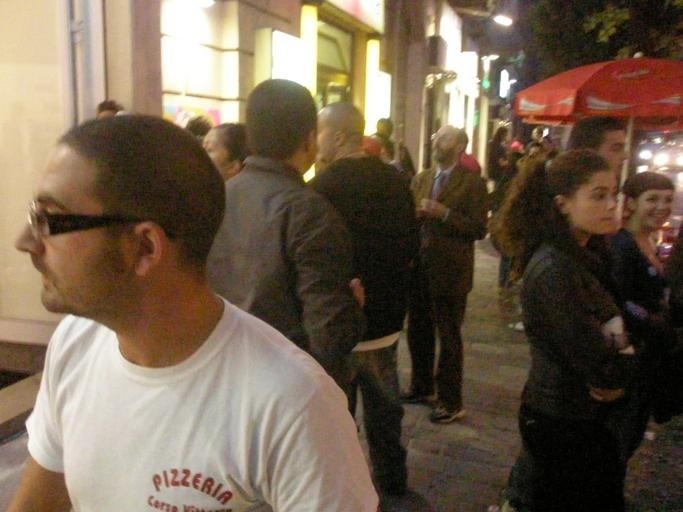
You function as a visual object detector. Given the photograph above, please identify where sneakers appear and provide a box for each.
[429,404,466,423]
[400,384,437,403]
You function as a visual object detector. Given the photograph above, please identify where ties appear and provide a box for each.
[432,172,444,200]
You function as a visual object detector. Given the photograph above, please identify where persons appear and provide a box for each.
[560,115,627,178]
[492,146,631,510]
[393,121,489,426]
[597,171,675,454]
[0,115,384,512]
[199,80,367,413]
[301,98,423,494]
[91,97,554,298]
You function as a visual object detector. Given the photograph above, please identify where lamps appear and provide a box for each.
[493,15,514,28]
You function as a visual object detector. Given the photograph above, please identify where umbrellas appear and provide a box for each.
[510,48,683,220]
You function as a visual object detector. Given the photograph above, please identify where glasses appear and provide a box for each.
[27,200,179,241]
